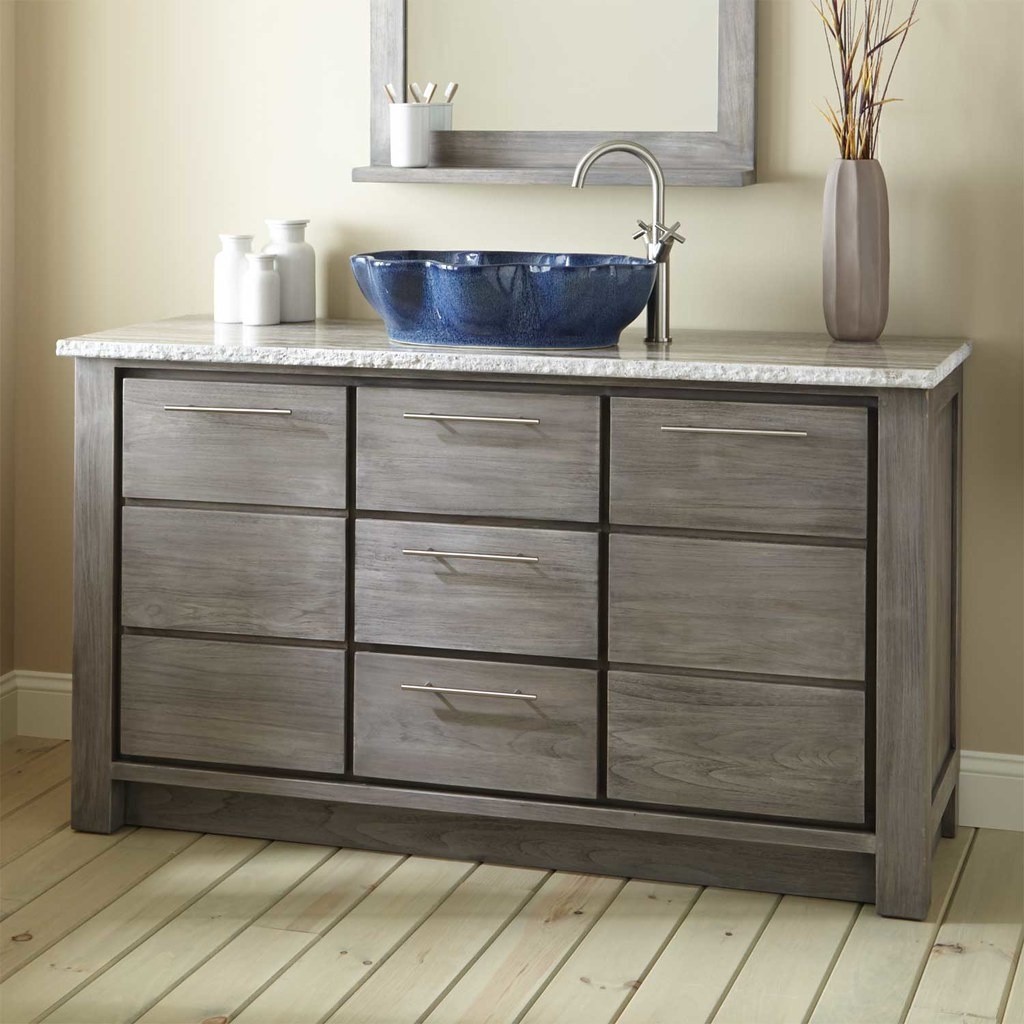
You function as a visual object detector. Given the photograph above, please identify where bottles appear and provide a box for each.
[260,219,316,323]
[213,234,254,323]
[239,253,281,326]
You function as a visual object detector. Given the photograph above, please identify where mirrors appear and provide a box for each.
[350,0,755,189]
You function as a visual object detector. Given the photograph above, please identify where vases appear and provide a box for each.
[823,158,890,341]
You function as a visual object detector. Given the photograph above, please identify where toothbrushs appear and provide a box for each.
[444,81,459,103]
[383,82,397,103]
[407,82,423,104]
[423,81,438,105]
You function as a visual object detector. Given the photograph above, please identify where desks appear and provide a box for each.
[54,312,975,919]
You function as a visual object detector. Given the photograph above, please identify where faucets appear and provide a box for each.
[568,138,685,345]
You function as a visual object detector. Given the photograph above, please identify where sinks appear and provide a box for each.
[348,250,659,349]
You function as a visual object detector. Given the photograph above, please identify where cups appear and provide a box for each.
[390,102,429,168]
[429,103,453,130]
